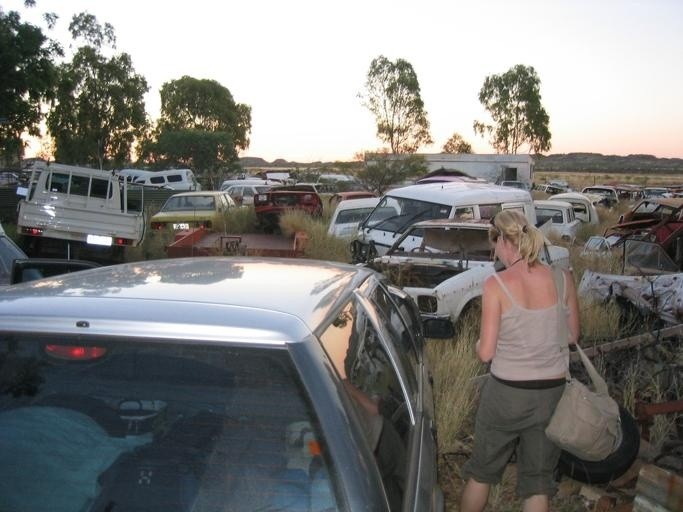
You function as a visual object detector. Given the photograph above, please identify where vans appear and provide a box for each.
[350,181,538,264]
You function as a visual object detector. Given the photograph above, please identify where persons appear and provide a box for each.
[343,378,406,511]
[460,210,580,512]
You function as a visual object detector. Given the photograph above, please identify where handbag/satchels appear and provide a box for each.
[545,378,622,462]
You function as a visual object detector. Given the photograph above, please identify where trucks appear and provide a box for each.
[15,160,147,265]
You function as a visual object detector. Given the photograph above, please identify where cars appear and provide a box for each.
[413,173,683,325]
[355,218,575,348]
[149,191,250,239]
[326,196,401,242]
[253,184,324,227]
[111,168,298,205]
[0,168,42,189]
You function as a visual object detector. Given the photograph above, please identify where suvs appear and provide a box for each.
[0,254,446,512]
